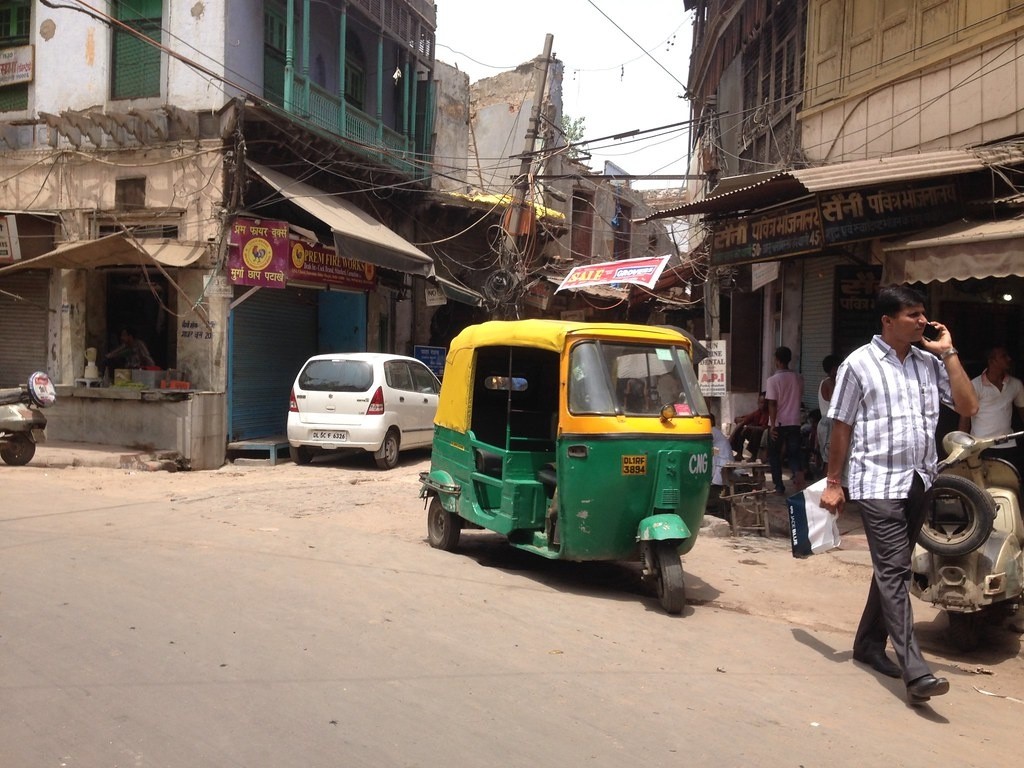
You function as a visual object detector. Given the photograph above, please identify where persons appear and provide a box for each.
[707,413,736,512]
[808,409,822,469]
[729,391,768,462]
[819,284,979,702]
[766,345,802,495]
[105,327,149,369]
[817,354,841,462]
[959,342,1024,468]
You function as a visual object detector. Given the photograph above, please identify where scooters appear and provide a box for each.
[0,371,57,467]
[907,428,1024,649]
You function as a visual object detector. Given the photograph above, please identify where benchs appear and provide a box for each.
[535,464,558,497]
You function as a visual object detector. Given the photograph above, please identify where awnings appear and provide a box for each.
[537,270,627,300]
[878,213,1024,287]
[0,233,210,273]
[414,274,483,307]
[244,159,436,278]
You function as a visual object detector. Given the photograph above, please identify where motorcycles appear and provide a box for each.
[413,318,720,618]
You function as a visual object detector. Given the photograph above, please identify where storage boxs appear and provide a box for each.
[113,368,133,385]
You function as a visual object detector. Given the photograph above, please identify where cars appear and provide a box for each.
[286,353,442,470]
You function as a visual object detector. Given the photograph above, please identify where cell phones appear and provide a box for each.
[922,322,941,341]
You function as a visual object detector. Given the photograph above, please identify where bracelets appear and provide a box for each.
[827,479,839,484]
[940,348,957,359]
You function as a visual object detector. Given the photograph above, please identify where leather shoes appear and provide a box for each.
[907,675,949,704]
[853,650,903,677]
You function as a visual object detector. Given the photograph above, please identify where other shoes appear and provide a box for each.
[773,489,784,496]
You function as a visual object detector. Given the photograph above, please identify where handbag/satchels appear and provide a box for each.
[786,477,841,559]
